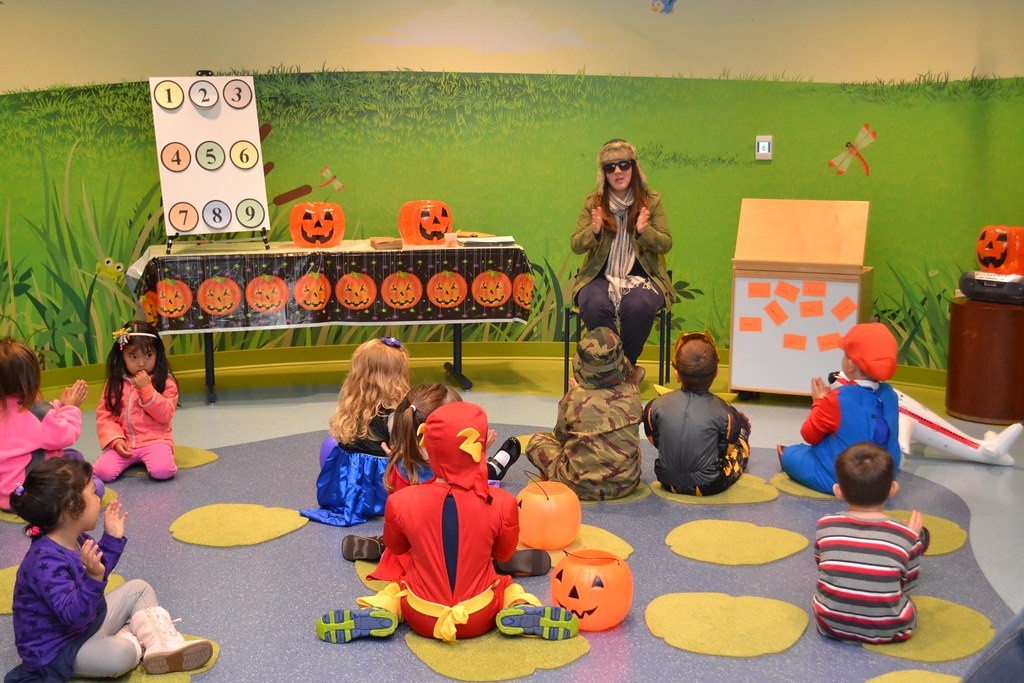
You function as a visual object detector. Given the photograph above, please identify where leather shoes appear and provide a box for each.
[488,437,521,481]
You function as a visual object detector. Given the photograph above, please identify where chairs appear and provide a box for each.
[563,270,673,398]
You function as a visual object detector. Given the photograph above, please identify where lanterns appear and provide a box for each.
[399,201,454,248]
[511,469,634,632]
[290,203,347,248]
[975,225,1024,274]
[139,264,535,327]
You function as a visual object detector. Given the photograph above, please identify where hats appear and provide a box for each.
[836,322,898,381]
[596,138,648,196]
[572,327,634,389]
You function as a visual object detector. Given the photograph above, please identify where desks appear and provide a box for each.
[944,298,1024,425]
[126,237,535,403]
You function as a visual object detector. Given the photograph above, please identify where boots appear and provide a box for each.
[130,606,213,674]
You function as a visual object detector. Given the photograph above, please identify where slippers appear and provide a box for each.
[623,355,646,388]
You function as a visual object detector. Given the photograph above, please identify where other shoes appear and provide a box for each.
[341,535,386,561]
[493,549,551,576]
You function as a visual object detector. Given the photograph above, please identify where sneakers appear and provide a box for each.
[495,604,580,641]
[316,607,398,644]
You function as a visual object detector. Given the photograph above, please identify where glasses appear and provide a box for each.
[602,160,634,174]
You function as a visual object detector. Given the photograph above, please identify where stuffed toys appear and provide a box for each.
[826,371,1024,471]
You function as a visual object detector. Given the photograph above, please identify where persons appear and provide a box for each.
[1,337,106,513]
[526,325,646,501]
[341,383,552,576]
[91,319,180,482]
[316,402,579,645]
[643,329,752,496]
[777,322,902,497]
[4,456,213,683]
[299,336,523,528]
[571,139,677,387]
[813,443,930,644]
